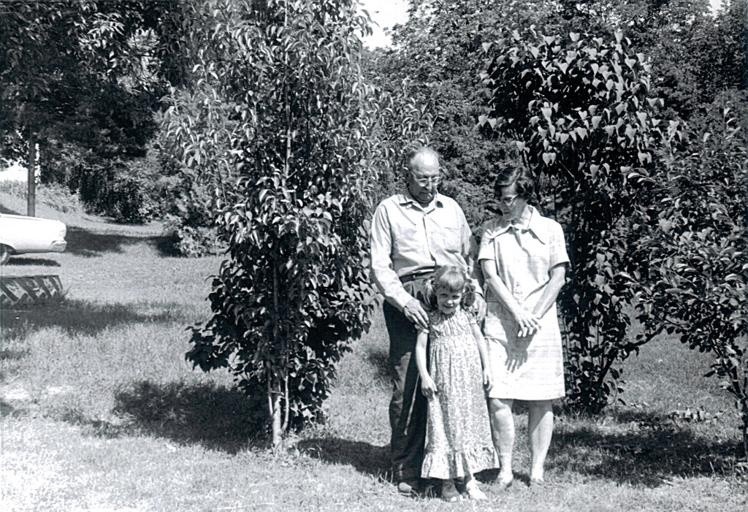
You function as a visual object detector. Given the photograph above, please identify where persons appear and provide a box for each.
[477,166,574,490]
[372,147,488,495]
[414,266,500,501]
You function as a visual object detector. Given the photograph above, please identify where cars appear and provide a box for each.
[0,208,67,267]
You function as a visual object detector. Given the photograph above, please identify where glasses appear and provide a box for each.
[495,193,518,204]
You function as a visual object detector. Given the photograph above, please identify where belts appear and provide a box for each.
[399,273,433,282]
